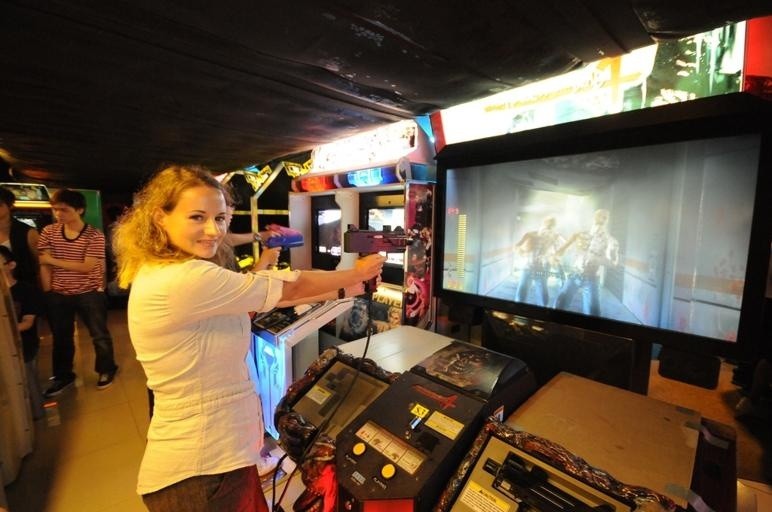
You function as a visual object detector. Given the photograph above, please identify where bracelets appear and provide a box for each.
[337,287,346,299]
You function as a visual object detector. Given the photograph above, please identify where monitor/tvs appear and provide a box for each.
[311,207,342,258]
[360,205,404,269]
[432,109,772,358]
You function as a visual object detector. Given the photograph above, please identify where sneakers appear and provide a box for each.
[96,369,117,390]
[43,377,78,397]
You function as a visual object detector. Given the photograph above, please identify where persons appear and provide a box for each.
[513,213,565,308]
[0,246,40,422]
[1,187,40,318]
[36,189,119,398]
[148,182,284,424]
[106,160,386,512]
[552,209,618,317]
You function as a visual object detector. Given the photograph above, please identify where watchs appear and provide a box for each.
[253,232,261,242]
